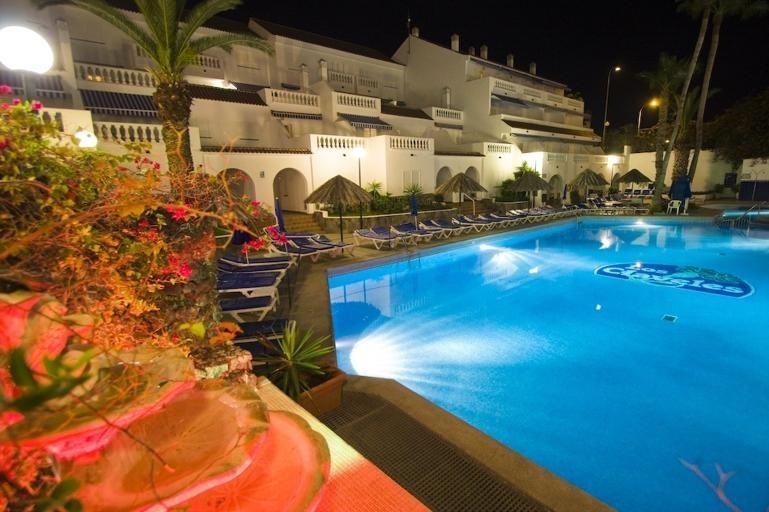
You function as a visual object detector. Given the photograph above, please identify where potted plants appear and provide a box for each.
[253,319,348,417]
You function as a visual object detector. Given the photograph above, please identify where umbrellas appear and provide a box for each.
[507,170,553,213]
[615,169,651,200]
[305,175,374,254]
[434,173,488,218]
[569,168,609,204]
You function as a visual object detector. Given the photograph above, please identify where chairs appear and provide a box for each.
[666,200,682,216]
[575,190,655,216]
[218,225,357,369]
[479,204,581,228]
[356,216,496,251]
